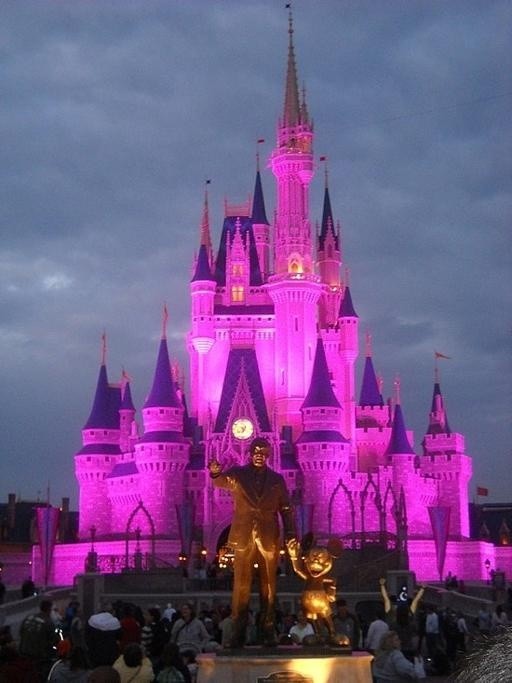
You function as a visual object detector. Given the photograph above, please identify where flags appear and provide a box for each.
[436,353,450,359]
[477,487,488,496]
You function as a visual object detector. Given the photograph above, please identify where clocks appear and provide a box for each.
[232,419,253,439]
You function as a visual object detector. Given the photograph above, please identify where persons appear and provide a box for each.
[208,438,297,647]
[1,565,234,683]
[234,565,511,681]
